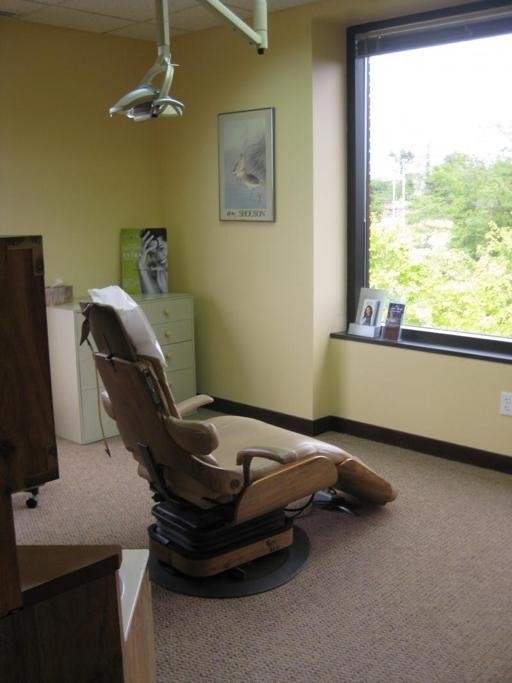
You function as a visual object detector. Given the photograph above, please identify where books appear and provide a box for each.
[383,303,405,342]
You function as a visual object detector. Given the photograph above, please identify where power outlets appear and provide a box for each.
[500,392,512,417]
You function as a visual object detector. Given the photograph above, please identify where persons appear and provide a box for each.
[362,305,373,326]
[138,228,168,294]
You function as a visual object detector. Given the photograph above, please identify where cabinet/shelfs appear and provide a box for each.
[47,295,197,445]
[2,234,154,683]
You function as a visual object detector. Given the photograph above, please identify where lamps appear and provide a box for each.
[108,44,187,123]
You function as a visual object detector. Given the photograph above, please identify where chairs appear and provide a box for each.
[78,300,398,599]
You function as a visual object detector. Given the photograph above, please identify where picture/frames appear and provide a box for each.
[217,107,276,222]
